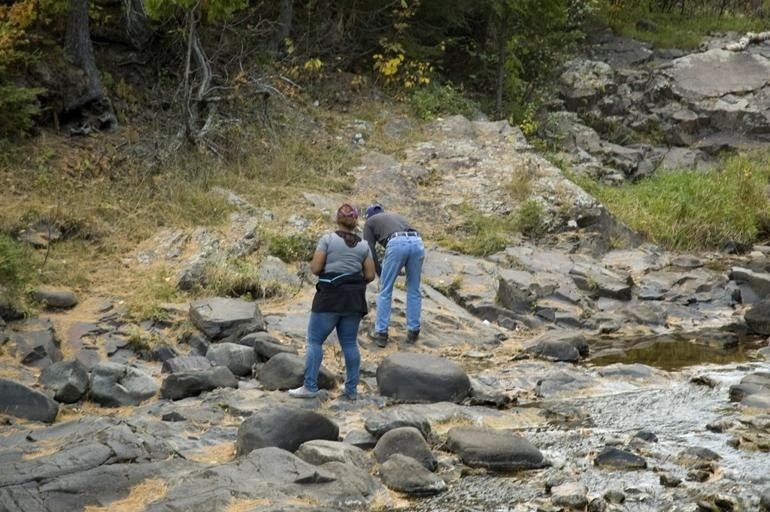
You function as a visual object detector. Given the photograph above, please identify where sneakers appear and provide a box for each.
[366,327,390,348]
[339,382,357,400]
[286,385,320,398]
[406,329,421,343]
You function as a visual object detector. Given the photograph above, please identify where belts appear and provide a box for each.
[384,232,420,248]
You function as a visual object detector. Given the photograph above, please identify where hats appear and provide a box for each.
[334,203,358,218]
[364,204,384,221]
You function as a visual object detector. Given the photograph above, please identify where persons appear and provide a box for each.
[288,203,376,400]
[361,203,425,348]
